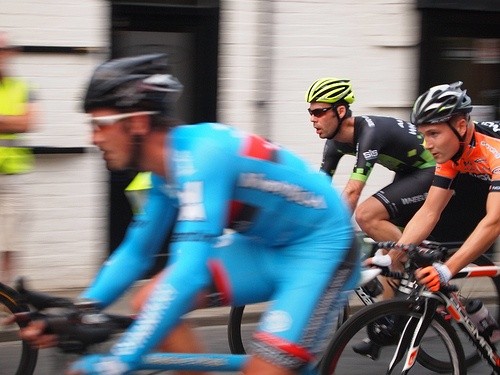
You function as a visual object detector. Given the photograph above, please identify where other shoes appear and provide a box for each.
[352,338,381,360]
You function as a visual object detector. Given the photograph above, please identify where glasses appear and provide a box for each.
[86,110,161,131]
[307,106,336,117]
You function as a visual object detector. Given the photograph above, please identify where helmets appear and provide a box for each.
[306,77,356,104]
[410,80,473,125]
[84,53,184,114]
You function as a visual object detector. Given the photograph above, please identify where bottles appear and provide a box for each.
[466,297,500,342]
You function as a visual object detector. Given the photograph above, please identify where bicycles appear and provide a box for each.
[0,232,500,375]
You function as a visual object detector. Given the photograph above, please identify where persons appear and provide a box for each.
[306,76,462,359]
[360,81,500,291]
[1,52,355,374]
[1,32,38,313]
